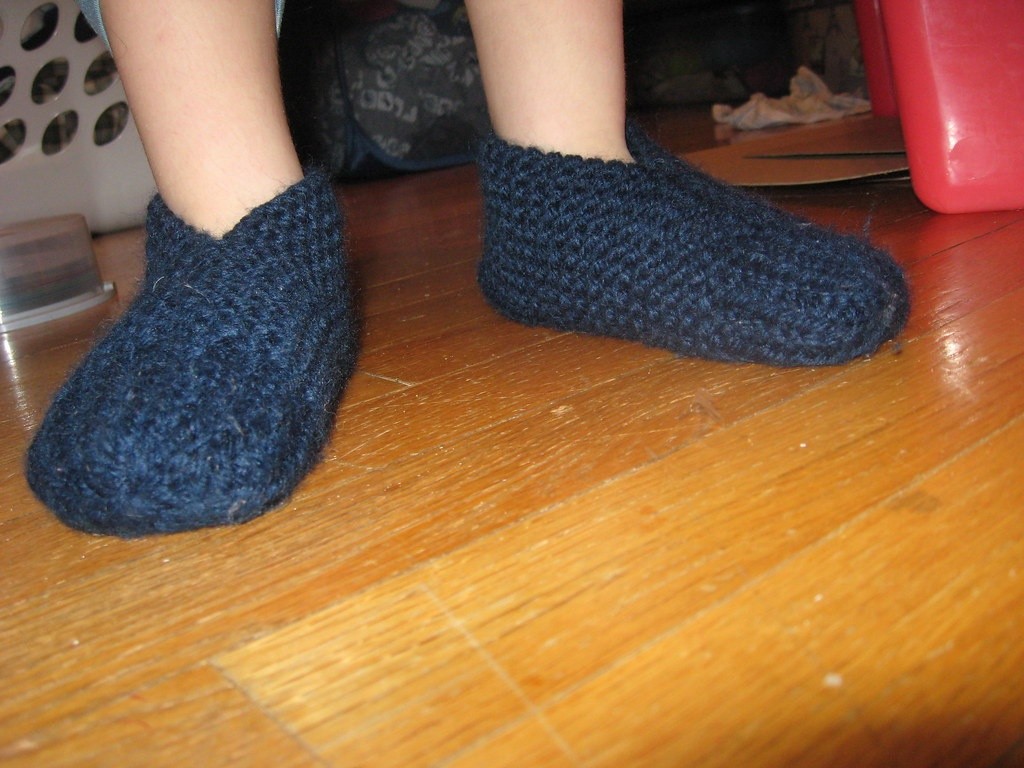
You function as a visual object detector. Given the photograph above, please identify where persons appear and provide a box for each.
[26,0,908,538]
